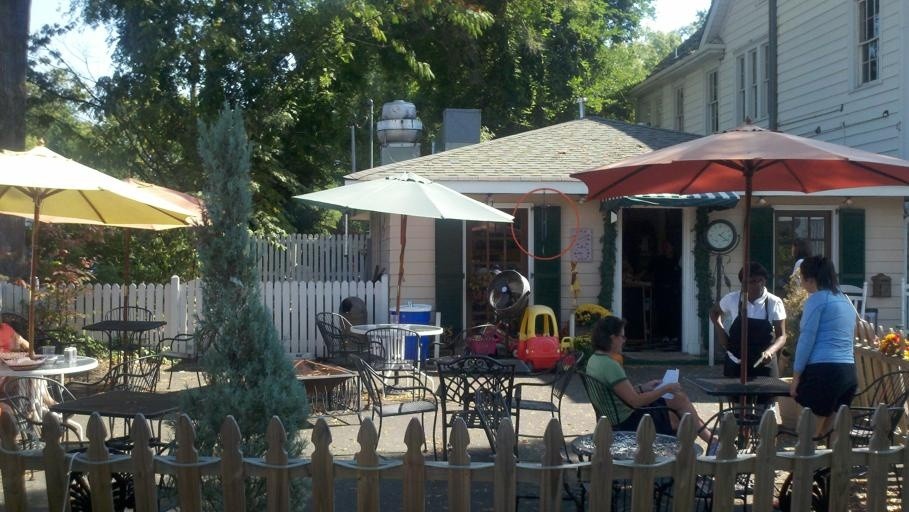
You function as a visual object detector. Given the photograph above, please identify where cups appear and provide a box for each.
[42,346,56,360]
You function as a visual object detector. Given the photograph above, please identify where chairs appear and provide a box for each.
[839,281,868,321]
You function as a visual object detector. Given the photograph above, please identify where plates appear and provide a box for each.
[0,356,47,370]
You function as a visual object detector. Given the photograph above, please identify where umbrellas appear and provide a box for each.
[288,170,516,326]
[568,122,909,448]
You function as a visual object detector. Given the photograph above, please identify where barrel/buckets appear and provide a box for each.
[390,300,434,361]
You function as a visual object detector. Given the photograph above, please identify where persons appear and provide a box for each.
[583,313,719,446]
[778,235,812,379]
[708,259,788,451]
[788,252,858,452]
[0,289,60,411]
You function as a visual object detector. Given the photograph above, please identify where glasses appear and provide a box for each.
[617,334,627,340]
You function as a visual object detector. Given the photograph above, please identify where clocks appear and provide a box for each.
[702,219,738,252]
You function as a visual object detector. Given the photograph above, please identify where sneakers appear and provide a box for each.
[708,437,738,456]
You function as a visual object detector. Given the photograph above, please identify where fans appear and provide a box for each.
[487,270,531,360]
